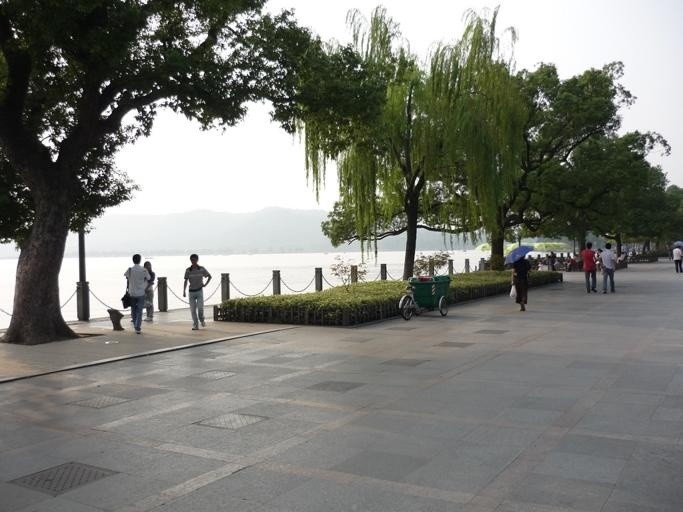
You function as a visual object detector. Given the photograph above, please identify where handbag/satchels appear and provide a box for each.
[121,293,130,309]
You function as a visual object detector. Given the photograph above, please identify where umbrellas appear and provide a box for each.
[504,246,534,264]
[673,241,683,247]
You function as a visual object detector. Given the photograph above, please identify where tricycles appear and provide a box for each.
[397,276,451,320]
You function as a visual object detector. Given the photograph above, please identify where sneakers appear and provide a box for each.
[202,322,205,326]
[192,327,197,330]
[591,288,597,292]
[587,289,590,292]
[144,317,152,321]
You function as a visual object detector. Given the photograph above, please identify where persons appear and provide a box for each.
[124,254,151,334]
[130,261,155,321]
[599,243,616,293]
[580,242,597,293]
[512,255,531,312]
[528,251,577,271]
[593,248,604,271]
[183,254,212,330]
[673,246,683,273]
[617,243,641,264]
